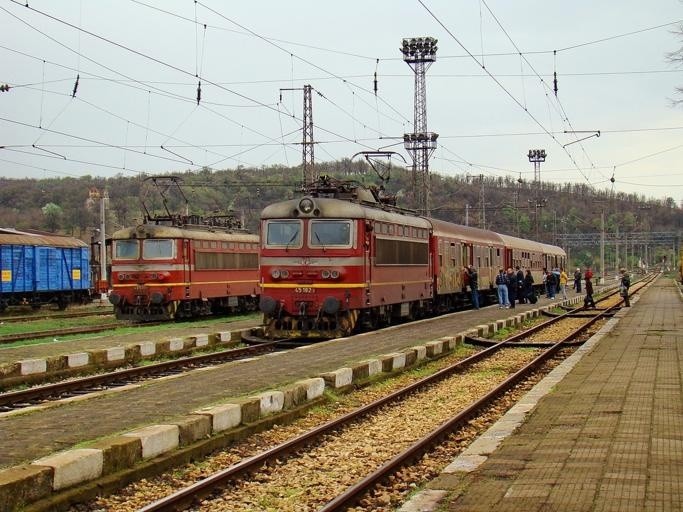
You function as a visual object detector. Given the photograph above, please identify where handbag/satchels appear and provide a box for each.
[585,296,594,307]
[620,287,628,296]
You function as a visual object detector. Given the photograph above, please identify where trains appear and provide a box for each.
[256,175,567,340]
[0,226,94,310]
[107,214,259,321]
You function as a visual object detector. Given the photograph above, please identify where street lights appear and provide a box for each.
[526,148,547,242]
[399,37,437,219]
[638,204,652,273]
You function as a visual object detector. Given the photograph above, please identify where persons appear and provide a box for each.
[618,267,630,307]
[463,266,596,311]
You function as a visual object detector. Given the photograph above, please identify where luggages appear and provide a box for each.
[527,292,537,304]
